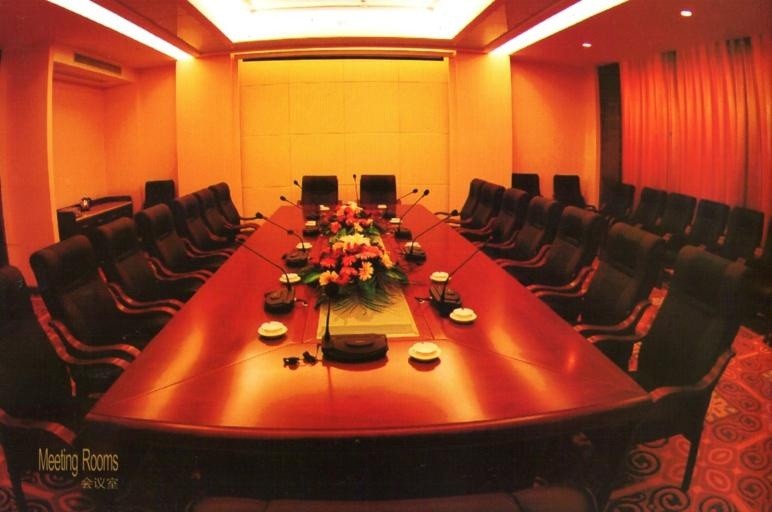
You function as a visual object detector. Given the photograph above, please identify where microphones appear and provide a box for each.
[229,233,294,314]
[352,174,366,210]
[394,189,430,240]
[294,180,322,219]
[255,211,308,267]
[379,188,418,221]
[403,208,459,263]
[427,227,502,311]
[280,195,320,237]
[322,283,387,364]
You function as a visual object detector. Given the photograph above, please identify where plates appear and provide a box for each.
[256,321,289,338]
[281,203,329,284]
[407,341,441,362]
[449,307,478,324]
[379,204,451,283]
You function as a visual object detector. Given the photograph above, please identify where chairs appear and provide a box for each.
[1,171,772,512]
